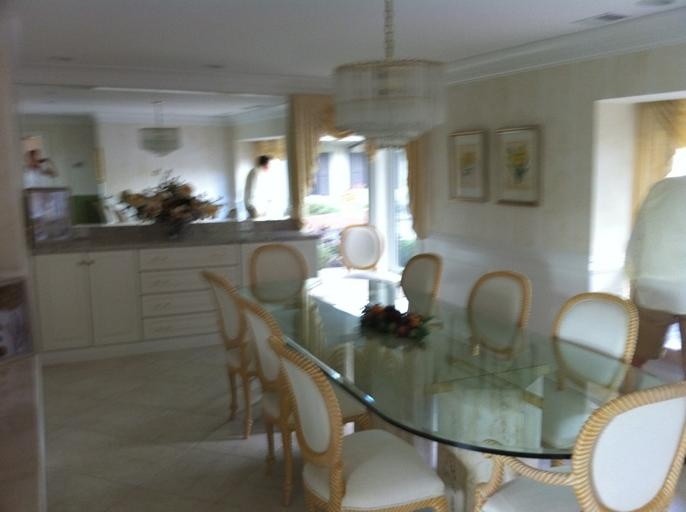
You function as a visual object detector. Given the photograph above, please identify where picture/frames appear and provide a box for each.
[447,123,543,207]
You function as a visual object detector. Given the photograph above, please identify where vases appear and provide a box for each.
[156,222,181,235]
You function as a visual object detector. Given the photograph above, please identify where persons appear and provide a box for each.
[618,176,685,366]
[241,155,274,217]
[23,147,57,190]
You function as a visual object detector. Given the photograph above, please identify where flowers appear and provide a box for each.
[116,176,223,222]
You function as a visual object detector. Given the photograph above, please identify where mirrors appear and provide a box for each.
[16,84,293,228]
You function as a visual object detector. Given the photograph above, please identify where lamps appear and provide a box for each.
[333,1,449,149]
[136,99,181,158]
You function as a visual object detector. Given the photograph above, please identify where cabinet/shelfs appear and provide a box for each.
[31,240,319,364]
